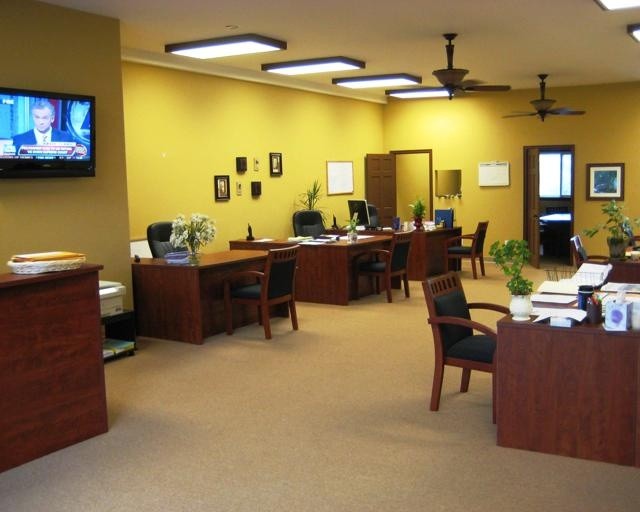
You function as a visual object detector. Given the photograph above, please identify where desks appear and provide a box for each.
[1,263,112,476]
[326,224,463,281]
[600,252,640,284]
[495,284,640,469]
[229,239,403,309]
[129,249,290,347]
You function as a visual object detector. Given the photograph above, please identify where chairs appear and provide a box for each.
[223,243,301,340]
[351,229,414,304]
[292,209,328,238]
[620,222,640,252]
[443,219,489,280]
[146,221,198,257]
[418,272,513,427]
[367,204,380,228]
[569,232,610,270]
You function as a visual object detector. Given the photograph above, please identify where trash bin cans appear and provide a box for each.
[100,310,136,353]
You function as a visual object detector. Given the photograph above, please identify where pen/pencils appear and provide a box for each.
[587,292,610,305]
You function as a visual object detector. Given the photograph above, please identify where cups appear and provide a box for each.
[586,303,603,323]
[630,251,640,261]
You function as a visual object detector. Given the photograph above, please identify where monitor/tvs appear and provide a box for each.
[0,87,96,178]
[348,200,371,226]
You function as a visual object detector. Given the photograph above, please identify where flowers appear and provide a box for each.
[169,214,216,256]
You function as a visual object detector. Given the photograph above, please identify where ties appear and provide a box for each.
[41,134,49,146]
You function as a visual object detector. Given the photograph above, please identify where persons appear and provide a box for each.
[13,101,78,159]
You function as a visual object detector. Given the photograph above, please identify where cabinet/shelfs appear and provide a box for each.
[101,308,135,365]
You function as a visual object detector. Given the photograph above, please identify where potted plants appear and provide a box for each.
[488,237,535,323]
[407,198,429,232]
[343,219,359,240]
[583,199,634,262]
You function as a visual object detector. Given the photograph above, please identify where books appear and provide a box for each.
[12,250,87,263]
[102,325,136,359]
[288,233,340,246]
[526,263,640,333]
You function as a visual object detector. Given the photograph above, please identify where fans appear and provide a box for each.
[502,75,587,122]
[406,34,512,96]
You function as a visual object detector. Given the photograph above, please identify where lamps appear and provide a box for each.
[163,32,289,61]
[331,73,423,90]
[626,22,639,43]
[260,55,369,78]
[383,86,457,100]
[593,0,637,11]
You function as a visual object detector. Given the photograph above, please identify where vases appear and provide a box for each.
[186,241,201,264]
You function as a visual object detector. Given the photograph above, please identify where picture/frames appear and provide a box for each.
[269,152,282,176]
[212,174,231,202]
[585,163,625,202]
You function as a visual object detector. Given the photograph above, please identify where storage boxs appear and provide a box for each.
[98,283,127,318]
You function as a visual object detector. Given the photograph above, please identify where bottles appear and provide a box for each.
[579,285,594,312]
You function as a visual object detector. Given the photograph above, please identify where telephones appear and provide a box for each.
[246,225,255,241]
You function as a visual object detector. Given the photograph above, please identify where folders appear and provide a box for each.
[436,209,454,229]
